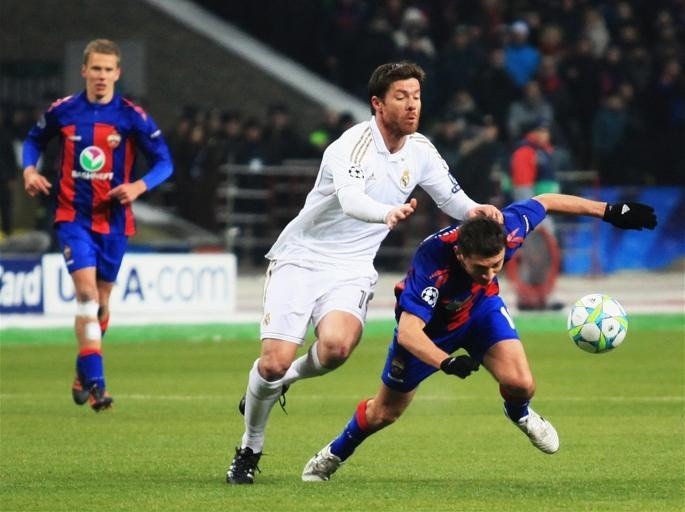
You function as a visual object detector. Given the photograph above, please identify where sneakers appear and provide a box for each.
[225,446,265,486]
[237,380,290,418]
[300,438,347,485]
[501,399,562,456]
[88,381,113,410]
[70,374,89,405]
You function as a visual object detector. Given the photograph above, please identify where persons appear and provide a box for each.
[225,62,504,485]
[303,192,659,484]
[509,116,574,313]
[0,1,683,277]
[22,39,174,411]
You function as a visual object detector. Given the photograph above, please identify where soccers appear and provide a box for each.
[568,292,628,356]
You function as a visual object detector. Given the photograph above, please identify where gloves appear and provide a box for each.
[439,354,474,380]
[602,199,658,233]
